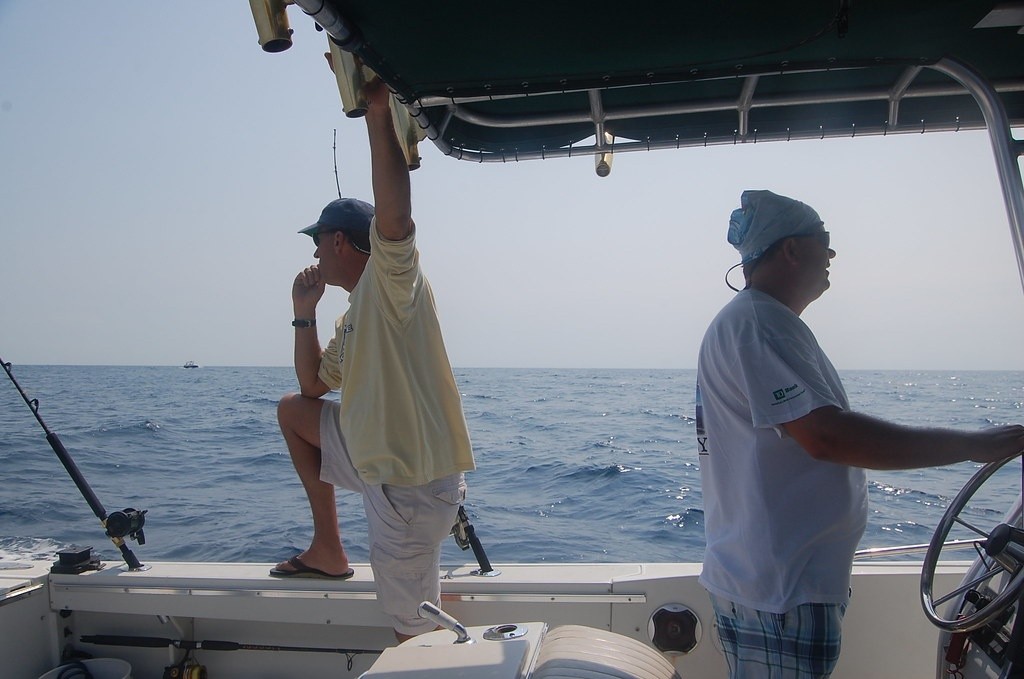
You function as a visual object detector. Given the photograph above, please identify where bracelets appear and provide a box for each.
[292,318,316,327]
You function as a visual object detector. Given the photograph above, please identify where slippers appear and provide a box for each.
[270,555,356,579]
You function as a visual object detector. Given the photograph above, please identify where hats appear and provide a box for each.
[297,196,376,235]
[727,189,823,259]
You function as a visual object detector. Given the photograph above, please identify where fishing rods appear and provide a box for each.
[0,359,148,572]
[79,634,384,655]
[333,130,493,573]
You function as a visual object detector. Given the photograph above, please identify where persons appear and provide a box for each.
[268,77,477,644]
[691,189,1023,679]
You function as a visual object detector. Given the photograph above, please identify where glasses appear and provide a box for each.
[791,232,831,247]
[312,228,339,247]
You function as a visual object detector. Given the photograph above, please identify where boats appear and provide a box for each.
[183,361,199,368]
